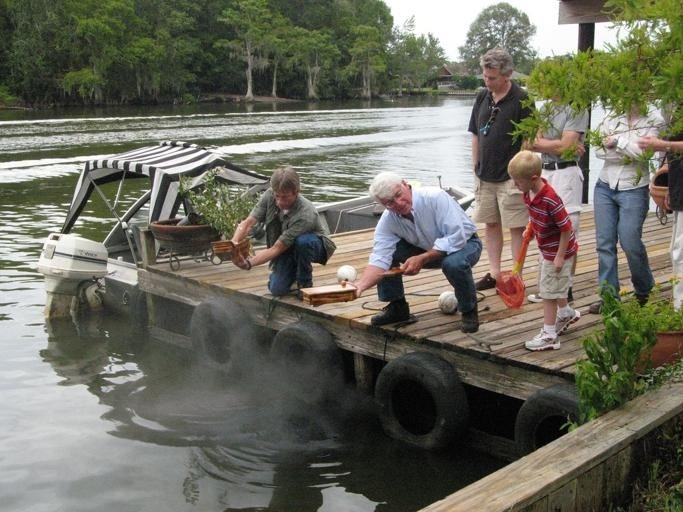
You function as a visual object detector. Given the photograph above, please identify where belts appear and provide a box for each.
[542,159,578,170]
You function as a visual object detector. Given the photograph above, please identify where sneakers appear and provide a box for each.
[527,292,544,303]
[555,308,583,337]
[296,280,314,300]
[523,328,561,351]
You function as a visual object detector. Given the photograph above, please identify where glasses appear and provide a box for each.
[381,183,405,209]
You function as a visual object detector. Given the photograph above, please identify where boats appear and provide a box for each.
[36,139,475,340]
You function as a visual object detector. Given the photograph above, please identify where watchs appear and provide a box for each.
[246,260,252,271]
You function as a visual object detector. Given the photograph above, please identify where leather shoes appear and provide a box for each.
[460,304,479,334]
[633,279,656,307]
[475,273,502,290]
[368,301,411,327]
[588,297,616,314]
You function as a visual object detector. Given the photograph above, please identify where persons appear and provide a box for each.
[589,77,665,315]
[228,167,337,300]
[468,49,540,295]
[527,64,590,307]
[495,150,579,351]
[637,63,683,314]
[340,170,483,333]
